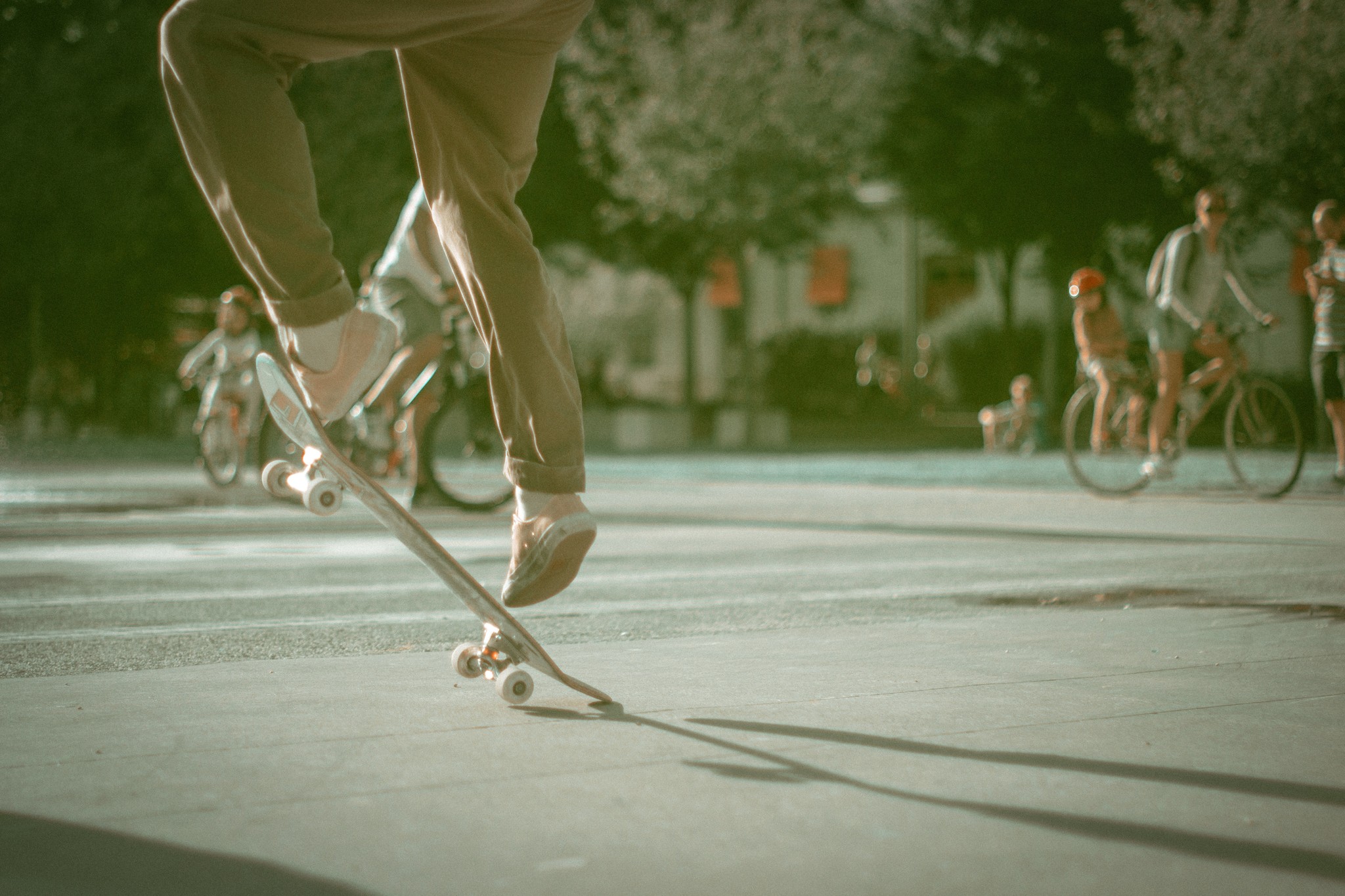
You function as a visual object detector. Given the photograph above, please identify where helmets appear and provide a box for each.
[1067,266,1109,293]
[222,285,262,317]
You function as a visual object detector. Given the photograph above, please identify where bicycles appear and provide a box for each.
[186,366,240,489]
[1062,316,1307,502]
[253,314,515,510]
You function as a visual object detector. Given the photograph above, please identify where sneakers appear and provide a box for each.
[501,493,595,608]
[287,307,398,423]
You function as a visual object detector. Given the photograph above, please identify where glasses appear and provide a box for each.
[1203,205,1229,215]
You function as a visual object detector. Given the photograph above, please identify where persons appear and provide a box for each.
[1302,200,1345,486]
[343,168,464,507]
[178,288,269,451]
[1068,268,1148,458]
[155,0,601,608]
[1139,187,1273,482]
[978,375,1045,456]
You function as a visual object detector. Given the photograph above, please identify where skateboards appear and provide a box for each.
[255,352,612,706]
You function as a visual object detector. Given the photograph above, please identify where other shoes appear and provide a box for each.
[1138,452,1174,481]
[1176,385,1212,413]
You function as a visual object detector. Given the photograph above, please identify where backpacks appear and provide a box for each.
[1145,224,1228,300]
